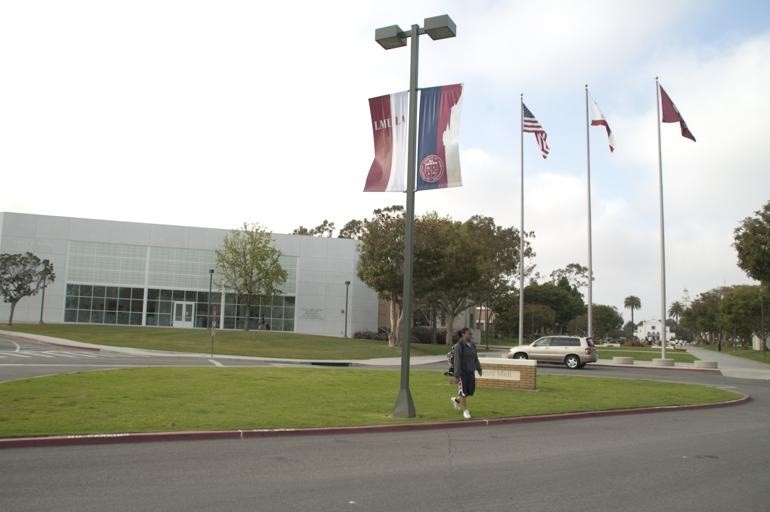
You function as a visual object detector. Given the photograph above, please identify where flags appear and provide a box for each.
[588,94,618,154]
[361,89,410,194]
[521,103,550,161]
[413,83,464,193]
[658,83,697,144]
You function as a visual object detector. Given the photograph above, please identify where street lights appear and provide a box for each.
[718,292,726,351]
[344,280,351,338]
[206,268,214,330]
[374,13,460,422]
[40,259,50,324]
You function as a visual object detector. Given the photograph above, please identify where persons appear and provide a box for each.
[258,310,267,331]
[647,334,653,345]
[450,327,483,419]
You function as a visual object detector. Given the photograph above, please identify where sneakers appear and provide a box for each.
[451,397,459,408]
[463,409,471,419]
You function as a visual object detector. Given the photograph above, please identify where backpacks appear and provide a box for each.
[447,341,464,372]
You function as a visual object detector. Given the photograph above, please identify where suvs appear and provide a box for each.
[506,336,597,370]
